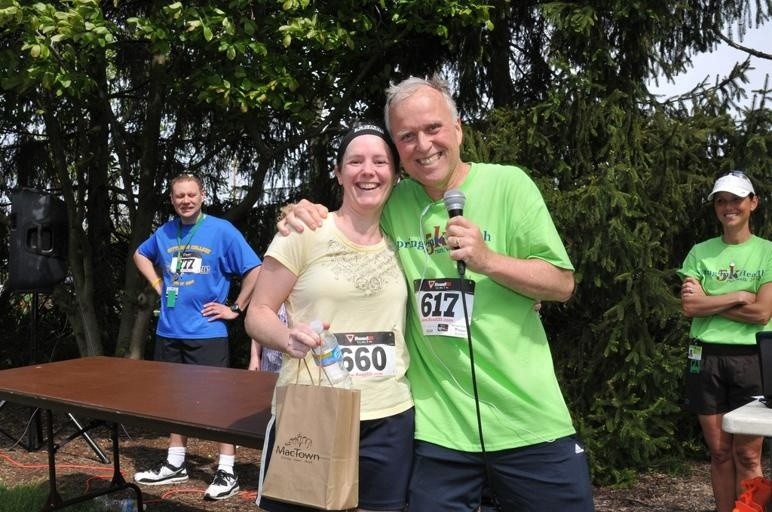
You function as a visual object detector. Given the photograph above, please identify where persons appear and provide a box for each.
[677,170,772,512]
[277,77,595,512]
[245,125,415,511]
[249,303,289,372]
[133,176,262,501]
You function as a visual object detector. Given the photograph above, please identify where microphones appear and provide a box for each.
[443,190,466,275]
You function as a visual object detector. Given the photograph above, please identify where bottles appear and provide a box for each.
[310,320,353,391]
[118,498,147,511]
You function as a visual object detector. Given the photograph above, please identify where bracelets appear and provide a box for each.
[152,277,162,287]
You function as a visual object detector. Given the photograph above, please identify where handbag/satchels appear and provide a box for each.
[259,331,360,512]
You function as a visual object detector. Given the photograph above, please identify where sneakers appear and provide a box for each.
[203,470,241,501]
[132,463,190,487]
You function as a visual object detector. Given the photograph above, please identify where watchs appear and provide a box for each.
[231,303,242,315]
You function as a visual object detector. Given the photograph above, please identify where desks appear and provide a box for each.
[721,395,772,436]
[0,355,279,511]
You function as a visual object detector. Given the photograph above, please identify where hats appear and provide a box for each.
[707,172,756,202]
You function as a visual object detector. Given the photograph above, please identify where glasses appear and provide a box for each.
[713,168,753,184]
